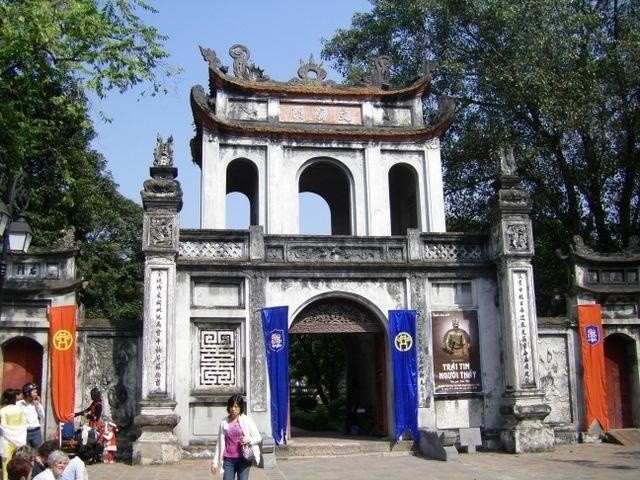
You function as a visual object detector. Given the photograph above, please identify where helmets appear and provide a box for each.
[23,382,39,391]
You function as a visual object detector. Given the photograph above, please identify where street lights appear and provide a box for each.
[1,196,39,319]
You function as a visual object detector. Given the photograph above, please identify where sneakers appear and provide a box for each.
[104,458,114,464]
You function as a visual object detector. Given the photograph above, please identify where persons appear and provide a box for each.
[211,394,261,480]
[441,317,470,357]
[0,382,120,480]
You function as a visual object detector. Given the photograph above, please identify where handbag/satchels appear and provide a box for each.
[242,443,261,465]
